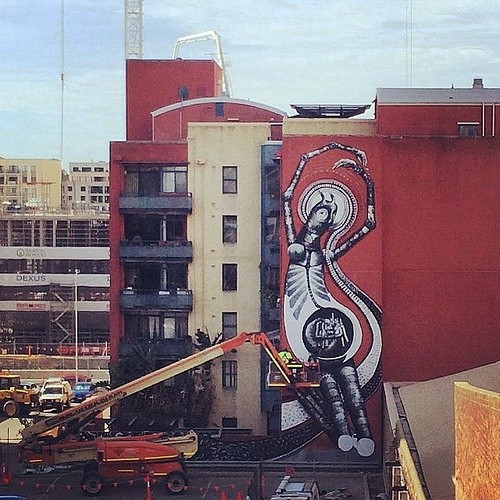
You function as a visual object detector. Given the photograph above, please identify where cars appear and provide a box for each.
[72,382,95,402]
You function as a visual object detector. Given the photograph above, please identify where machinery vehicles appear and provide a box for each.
[18,332,321,496]
[0,370,39,417]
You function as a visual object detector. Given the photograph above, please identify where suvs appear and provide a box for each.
[39,378,73,412]
